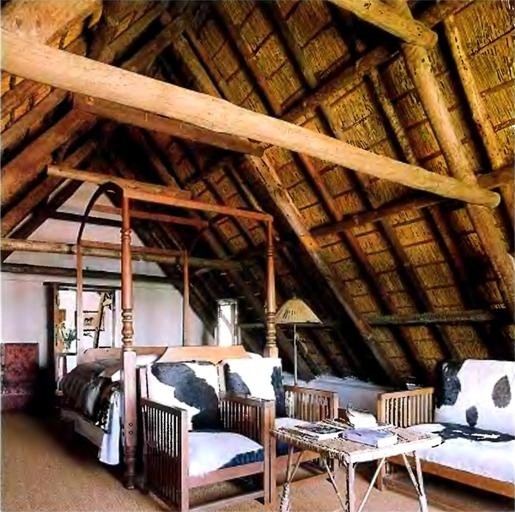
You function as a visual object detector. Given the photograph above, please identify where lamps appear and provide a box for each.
[275,294,325,386]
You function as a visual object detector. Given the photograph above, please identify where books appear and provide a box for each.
[342,428,400,449]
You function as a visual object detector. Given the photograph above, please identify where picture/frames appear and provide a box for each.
[73,309,105,331]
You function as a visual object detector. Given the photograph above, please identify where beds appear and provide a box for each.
[52,180,307,491]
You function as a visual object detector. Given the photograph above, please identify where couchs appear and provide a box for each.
[0,342,40,412]
[135,360,270,512]
[220,357,338,504]
[377,359,515,512]
